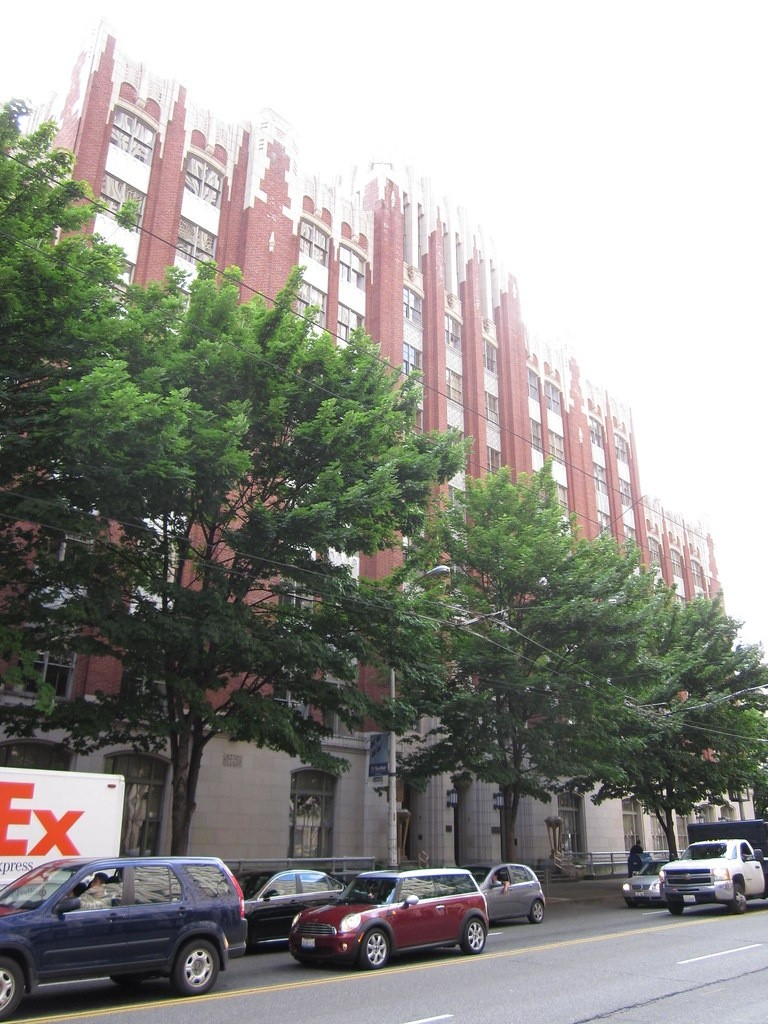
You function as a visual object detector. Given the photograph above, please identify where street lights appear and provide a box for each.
[387,562,453,869]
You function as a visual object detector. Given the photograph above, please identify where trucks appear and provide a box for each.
[0,764,125,907]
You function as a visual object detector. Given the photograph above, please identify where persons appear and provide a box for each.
[492,873,510,896]
[64,872,112,910]
[628,840,643,878]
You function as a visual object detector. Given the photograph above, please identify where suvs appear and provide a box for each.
[0,854,248,1023]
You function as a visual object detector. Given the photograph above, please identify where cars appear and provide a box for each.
[229,868,376,951]
[459,863,546,925]
[289,866,489,971]
[621,857,680,908]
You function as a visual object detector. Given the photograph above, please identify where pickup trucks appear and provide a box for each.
[658,839,768,917]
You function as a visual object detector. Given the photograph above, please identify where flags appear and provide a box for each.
[368,733,389,777]
